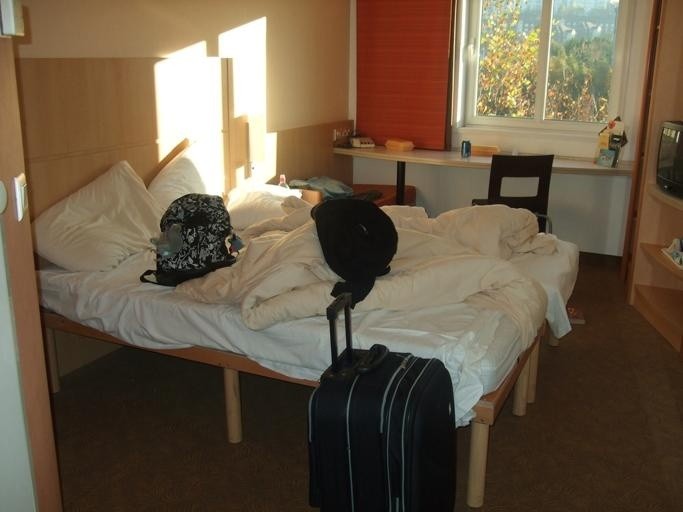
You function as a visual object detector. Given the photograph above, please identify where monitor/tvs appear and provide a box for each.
[655,119,683,198]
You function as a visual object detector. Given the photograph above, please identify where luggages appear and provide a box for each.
[306,293,456,512]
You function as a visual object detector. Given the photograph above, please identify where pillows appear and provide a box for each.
[149,155,207,211]
[30,158,161,274]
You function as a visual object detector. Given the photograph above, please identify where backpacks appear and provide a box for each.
[141,193,243,287]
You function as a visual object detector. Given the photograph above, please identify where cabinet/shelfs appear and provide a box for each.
[625,0,682,353]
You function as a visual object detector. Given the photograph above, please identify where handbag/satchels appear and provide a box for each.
[288,176,353,199]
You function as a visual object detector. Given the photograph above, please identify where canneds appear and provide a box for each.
[461,140,471,158]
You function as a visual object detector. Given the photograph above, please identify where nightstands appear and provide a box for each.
[348,182,416,209]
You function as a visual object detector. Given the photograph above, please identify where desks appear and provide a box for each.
[334,145,634,201]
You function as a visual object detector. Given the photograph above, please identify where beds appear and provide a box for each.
[35,204,578,508]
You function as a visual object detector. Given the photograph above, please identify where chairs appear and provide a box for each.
[470,154,555,234]
